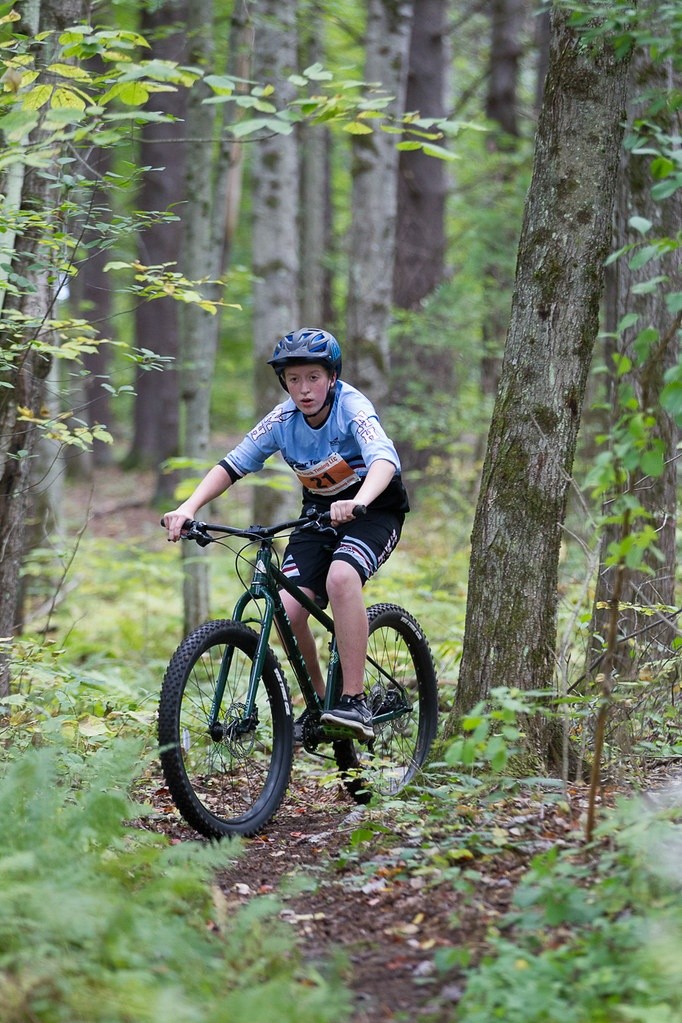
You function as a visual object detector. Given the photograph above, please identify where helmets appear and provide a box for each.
[266,327,342,379]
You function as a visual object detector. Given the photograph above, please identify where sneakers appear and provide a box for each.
[292,699,325,747]
[320,691,375,740]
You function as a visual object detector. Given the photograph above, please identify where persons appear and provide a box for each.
[164,328,411,739]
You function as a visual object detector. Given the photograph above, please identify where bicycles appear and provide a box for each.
[155,504,440,844]
